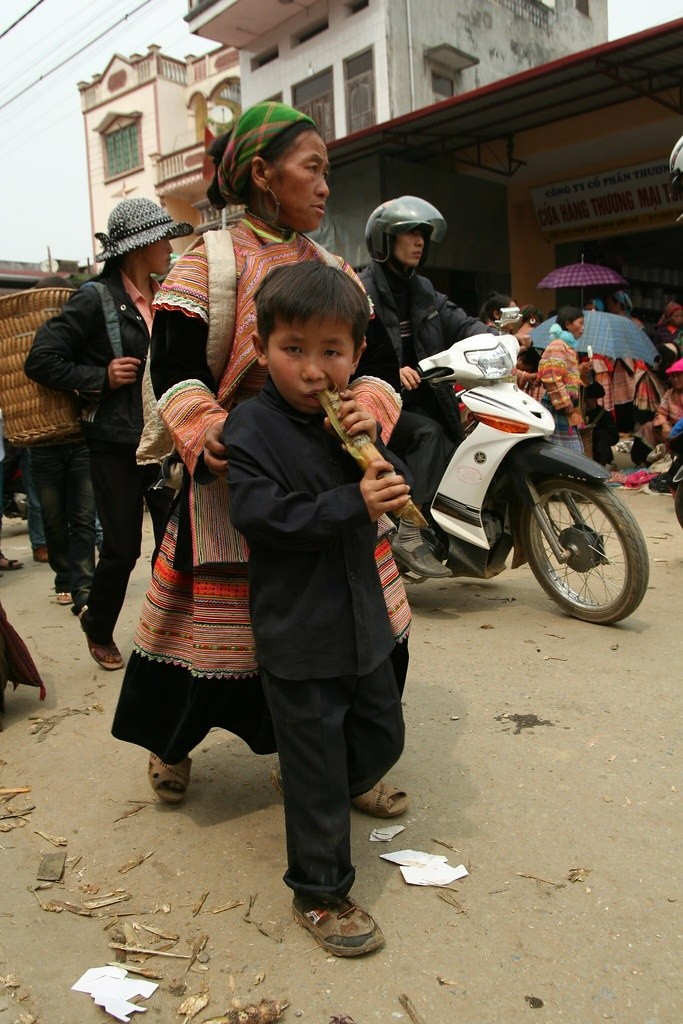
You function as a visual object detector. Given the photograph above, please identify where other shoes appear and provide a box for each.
[57,591,71,604]
[33,547,49,562]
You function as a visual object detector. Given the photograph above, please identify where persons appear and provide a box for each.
[476,292,538,390]
[0,408,23,569]
[586,290,683,464]
[513,304,543,394]
[21,197,194,672]
[536,307,593,503]
[108,103,411,819]
[26,278,96,617]
[24,448,50,562]
[355,195,531,579]
[217,261,409,956]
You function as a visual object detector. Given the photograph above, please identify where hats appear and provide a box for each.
[665,358,683,376]
[94,198,194,261]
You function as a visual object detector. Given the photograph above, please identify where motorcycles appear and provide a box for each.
[388,307,649,623]
[0,439,28,520]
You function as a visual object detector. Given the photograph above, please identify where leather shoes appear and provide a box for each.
[393,536,453,580]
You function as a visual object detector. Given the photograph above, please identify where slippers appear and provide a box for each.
[353,780,413,817]
[79,611,125,671]
[146,752,192,801]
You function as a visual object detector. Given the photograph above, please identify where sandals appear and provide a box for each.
[0,555,24,570]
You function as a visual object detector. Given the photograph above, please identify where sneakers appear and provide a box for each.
[293,895,384,958]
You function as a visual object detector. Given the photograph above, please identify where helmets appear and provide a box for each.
[366,195,434,261]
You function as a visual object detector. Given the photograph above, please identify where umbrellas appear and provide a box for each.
[529,310,660,368]
[534,253,628,306]
